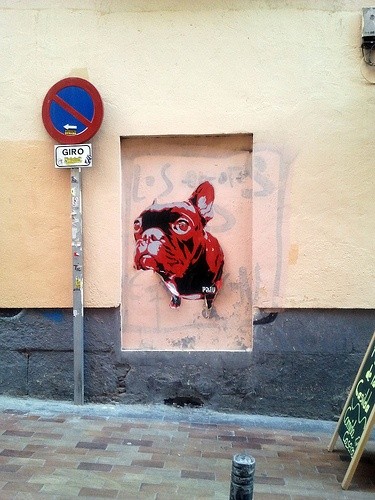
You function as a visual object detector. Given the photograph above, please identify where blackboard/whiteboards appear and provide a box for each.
[335,330,375,459]
